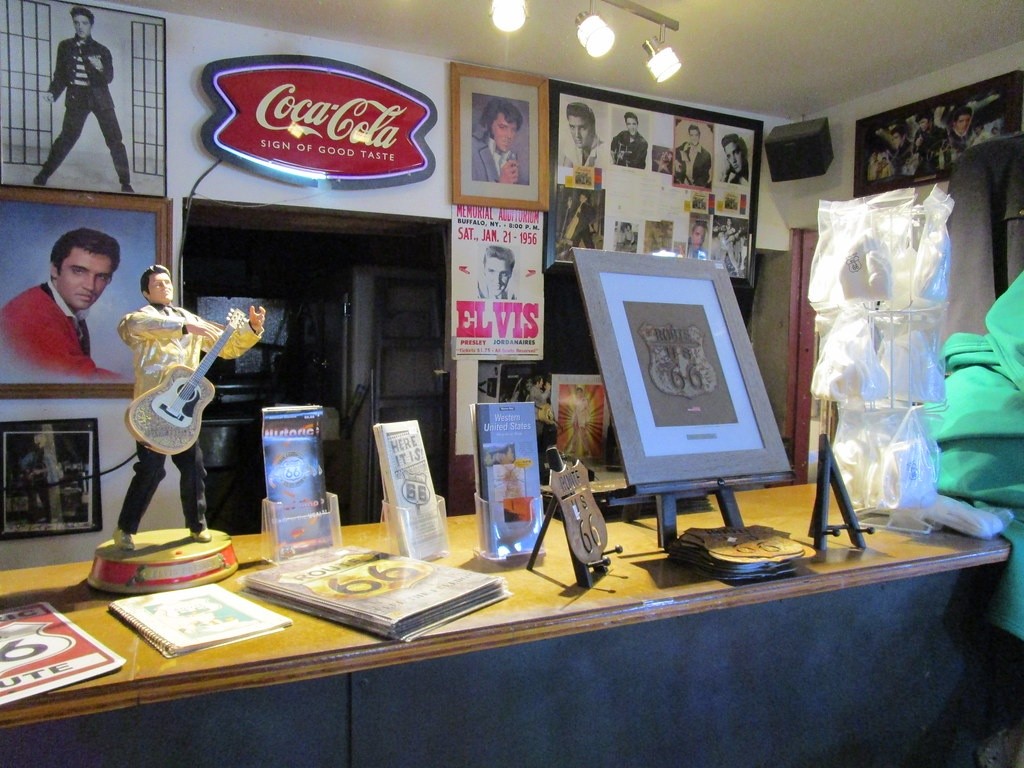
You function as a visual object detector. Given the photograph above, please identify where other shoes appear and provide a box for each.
[973,729,1008,768]
[122,185,134,192]
[34,174,48,185]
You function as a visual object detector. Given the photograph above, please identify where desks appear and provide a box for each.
[1,484,1011,768]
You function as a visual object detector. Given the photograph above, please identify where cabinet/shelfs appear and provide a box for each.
[817,204,950,537]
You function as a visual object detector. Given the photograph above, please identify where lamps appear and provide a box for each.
[644,10,682,82]
[572,0,616,58]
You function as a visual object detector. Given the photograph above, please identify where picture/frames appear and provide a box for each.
[450,61,550,211]
[0,0,168,198]
[543,78,764,289]
[854,70,1023,199]
[0,417,103,540]
[0,186,173,402]
[570,247,793,488]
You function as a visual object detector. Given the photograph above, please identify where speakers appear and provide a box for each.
[763,117,833,183]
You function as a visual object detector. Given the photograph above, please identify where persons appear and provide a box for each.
[110,264,268,551]
[477,244,518,301]
[867,149,890,176]
[714,217,744,275]
[33,6,136,194]
[911,108,948,151]
[674,124,711,188]
[560,191,598,250]
[688,222,709,259]
[567,387,593,457]
[717,133,749,184]
[510,374,557,435]
[615,222,637,254]
[949,106,973,146]
[558,102,604,168]
[973,119,1014,139]
[472,97,523,185]
[0,228,134,386]
[889,126,914,160]
[609,111,647,170]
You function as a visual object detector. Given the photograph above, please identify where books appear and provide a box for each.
[108,583,293,660]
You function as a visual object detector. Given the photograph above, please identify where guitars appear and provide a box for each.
[125,308,249,455]
